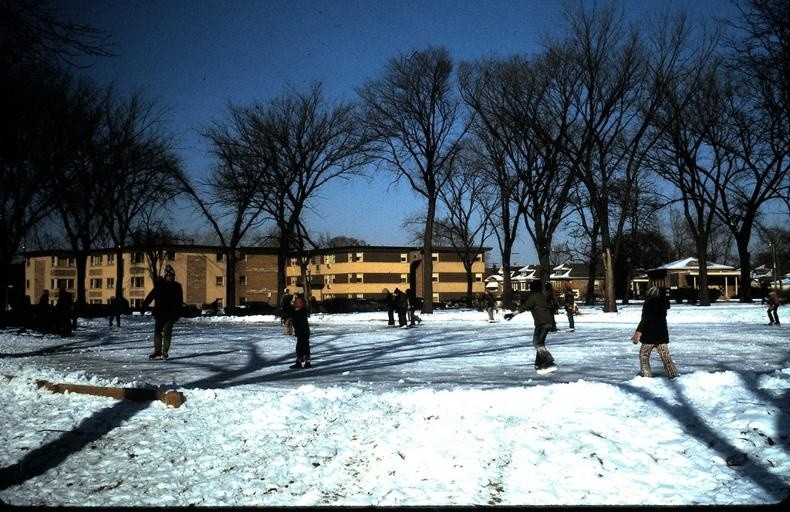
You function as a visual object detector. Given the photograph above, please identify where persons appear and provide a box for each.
[504,280,555,370]
[478,290,498,324]
[139,263,184,360]
[23,286,75,338]
[538,268,559,332]
[630,267,680,379]
[276,285,315,370]
[106,288,124,327]
[764,286,781,326]
[376,286,422,331]
[563,277,579,332]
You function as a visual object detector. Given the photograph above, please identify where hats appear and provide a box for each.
[647,286,660,297]
[163,264,175,277]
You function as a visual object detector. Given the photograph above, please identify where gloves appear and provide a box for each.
[140,307,145,316]
[504,314,514,321]
[631,332,642,345]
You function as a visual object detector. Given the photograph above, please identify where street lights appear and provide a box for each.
[768,240,778,287]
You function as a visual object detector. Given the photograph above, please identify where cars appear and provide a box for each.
[236,299,275,314]
[183,301,204,316]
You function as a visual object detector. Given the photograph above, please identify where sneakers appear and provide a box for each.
[163,352,168,358]
[303,362,311,369]
[149,351,162,358]
[289,361,301,369]
[537,361,555,371]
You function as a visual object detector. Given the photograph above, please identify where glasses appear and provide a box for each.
[168,274,176,277]
